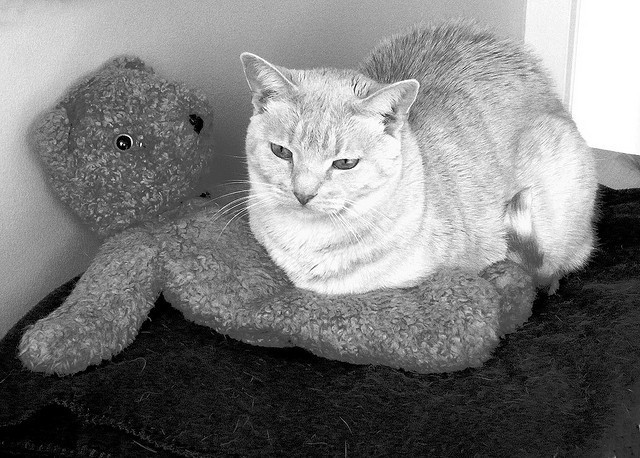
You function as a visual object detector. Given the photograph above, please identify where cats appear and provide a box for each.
[198,18,598,297]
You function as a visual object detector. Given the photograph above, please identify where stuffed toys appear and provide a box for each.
[16,54,535,377]
